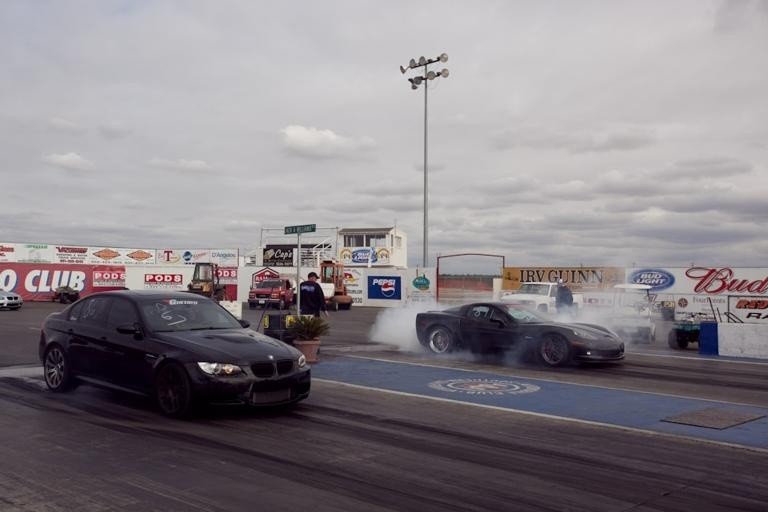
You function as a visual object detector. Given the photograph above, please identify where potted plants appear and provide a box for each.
[289,316,331,363]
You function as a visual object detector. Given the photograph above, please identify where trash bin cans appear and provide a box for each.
[698,321,718,355]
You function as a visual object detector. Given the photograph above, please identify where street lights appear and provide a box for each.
[395,52,448,268]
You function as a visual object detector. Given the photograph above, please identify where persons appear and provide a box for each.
[300,272,331,319]
[555,278,573,312]
[184,306,200,320]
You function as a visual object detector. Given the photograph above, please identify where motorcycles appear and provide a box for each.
[668,312,718,350]
[50,287,79,304]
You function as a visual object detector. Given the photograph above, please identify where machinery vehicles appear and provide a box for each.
[187,262,225,301]
[317,257,353,310]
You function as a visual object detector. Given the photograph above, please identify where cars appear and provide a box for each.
[38,289,311,420]
[0,289,23,310]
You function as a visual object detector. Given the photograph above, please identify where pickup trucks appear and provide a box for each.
[501,281,583,316]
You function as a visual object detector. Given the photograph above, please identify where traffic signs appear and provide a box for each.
[284,224,316,235]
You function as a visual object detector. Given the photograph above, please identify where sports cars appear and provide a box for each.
[416,303,624,367]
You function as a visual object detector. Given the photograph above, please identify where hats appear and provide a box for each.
[308,272,320,278]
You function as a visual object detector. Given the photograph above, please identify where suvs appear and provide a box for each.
[248,277,295,310]
